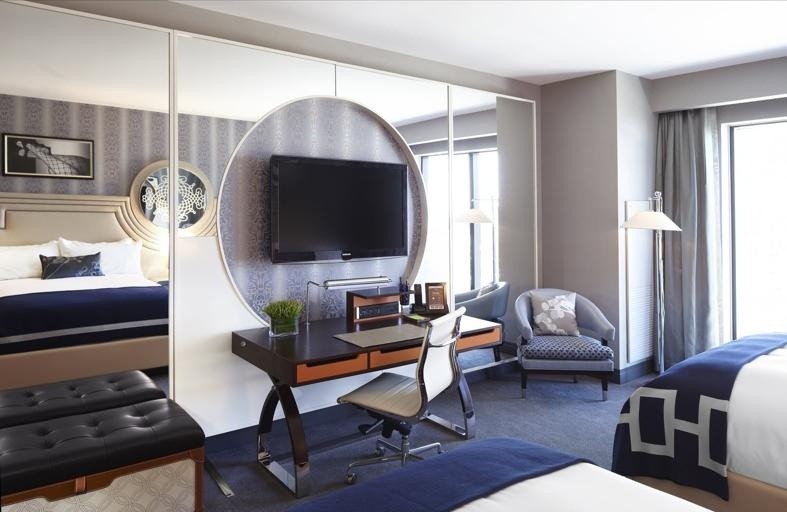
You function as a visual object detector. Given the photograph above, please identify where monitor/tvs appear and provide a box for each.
[269,155,408,264]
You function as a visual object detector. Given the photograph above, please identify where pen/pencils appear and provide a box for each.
[399,277,410,305]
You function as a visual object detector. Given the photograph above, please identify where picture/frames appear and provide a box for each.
[2,132,94,180]
[425,282,449,315]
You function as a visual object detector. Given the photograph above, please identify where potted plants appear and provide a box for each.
[261,298,305,338]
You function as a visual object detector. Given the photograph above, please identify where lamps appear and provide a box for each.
[304,274,393,327]
[456,195,497,283]
[619,190,684,375]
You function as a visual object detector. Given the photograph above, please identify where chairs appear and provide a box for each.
[455,280,511,362]
[336,305,468,488]
[514,287,616,402]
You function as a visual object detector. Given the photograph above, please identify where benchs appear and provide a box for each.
[0,397,207,512]
[1,369,166,431]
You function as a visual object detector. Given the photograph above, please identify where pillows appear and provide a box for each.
[0,238,60,280]
[529,289,582,337]
[475,282,498,297]
[38,252,104,280]
[57,236,144,277]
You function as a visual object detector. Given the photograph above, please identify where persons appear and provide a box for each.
[16,140,90,175]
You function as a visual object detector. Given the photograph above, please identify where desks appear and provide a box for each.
[231,306,503,500]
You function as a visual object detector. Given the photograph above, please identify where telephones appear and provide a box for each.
[413,282,449,315]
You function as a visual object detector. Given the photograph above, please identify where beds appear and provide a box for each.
[0,189,169,392]
[611,332,786,512]
[249,437,714,510]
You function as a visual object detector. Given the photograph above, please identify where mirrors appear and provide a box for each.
[336,61,453,236]
[450,84,541,372]
[172,29,335,237]
[0,0,172,426]
